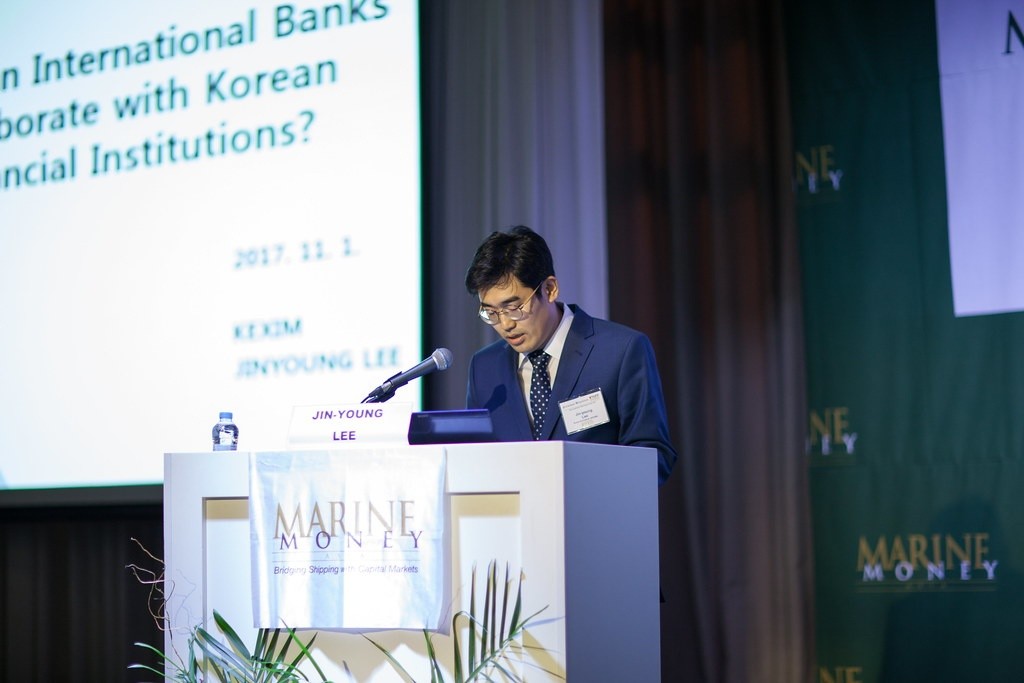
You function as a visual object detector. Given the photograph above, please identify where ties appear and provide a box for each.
[527,350,551,440]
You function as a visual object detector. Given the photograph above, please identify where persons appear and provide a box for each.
[465,226,680,496]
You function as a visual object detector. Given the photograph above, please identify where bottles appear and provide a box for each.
[212,412,239,451]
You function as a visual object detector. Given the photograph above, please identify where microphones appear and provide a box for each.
[368,349,454,397]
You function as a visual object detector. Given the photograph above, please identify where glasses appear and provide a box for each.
[478,282,542,325]
